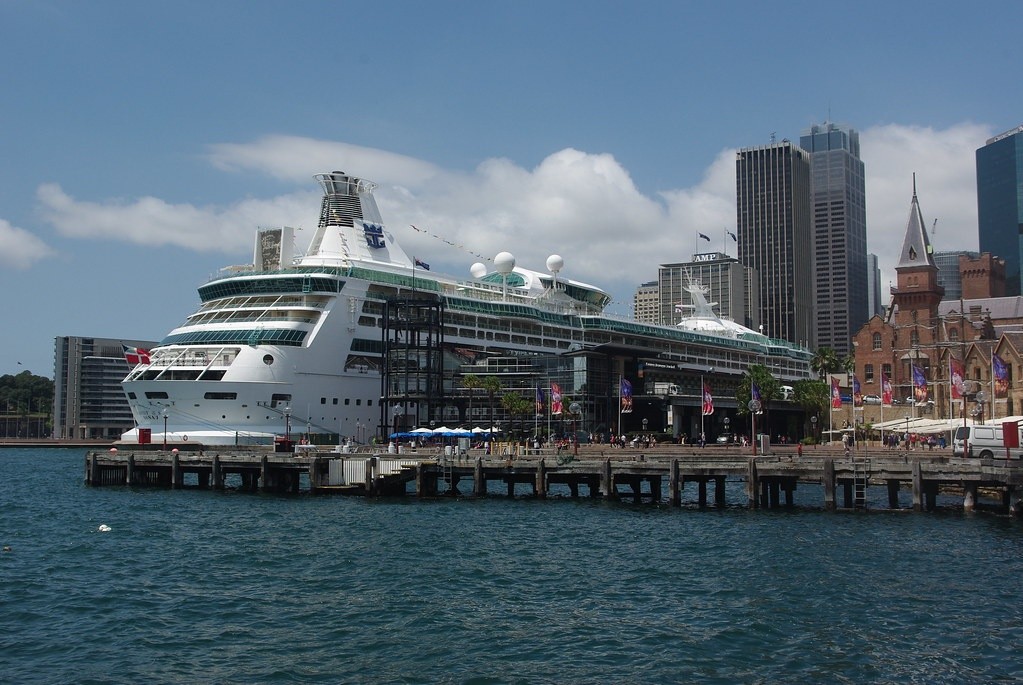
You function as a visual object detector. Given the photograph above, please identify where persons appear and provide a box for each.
[839,420,946,458]
[523,430,804,457]
[295,435,378,453]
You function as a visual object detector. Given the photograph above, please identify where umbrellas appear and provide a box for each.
[390,426,504,437]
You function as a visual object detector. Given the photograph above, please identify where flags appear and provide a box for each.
[122,345,151,365]
[415,257,429,270]
[699,233,710,241]
[729,232,737,241]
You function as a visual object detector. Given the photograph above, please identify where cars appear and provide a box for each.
[778,384,941,406]
[717,433,732,445]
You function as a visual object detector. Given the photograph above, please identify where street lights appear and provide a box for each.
[723,417,728,446]
[852,412,861,451]
[283,406,293,451]
[642,418,649,450]
[748,398,761,455]
[957,381,970,457]
[495,418,501,458]
[356,421,366,446]
[811,414,818,449]
[392,403,405,455]
[161,407,170,453]
[904,411,911,452]
[430,420,435,448]
[569,402,582,457]
[970,391,986,425]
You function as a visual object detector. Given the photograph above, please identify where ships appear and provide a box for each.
[114,167,812,457]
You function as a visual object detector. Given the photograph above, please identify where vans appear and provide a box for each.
[953,424,1023,462]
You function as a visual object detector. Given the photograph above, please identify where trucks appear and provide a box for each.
[643,381,683,396]
